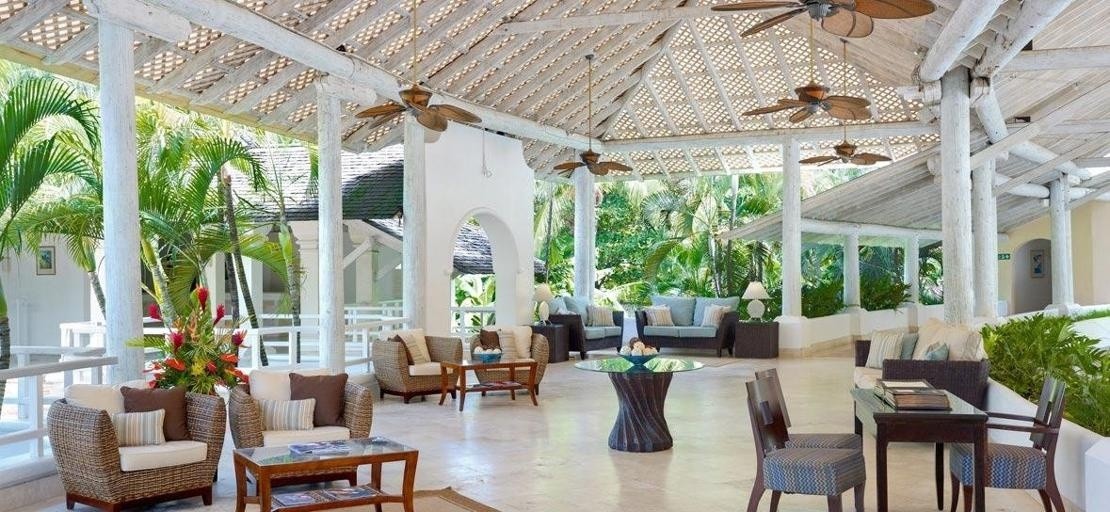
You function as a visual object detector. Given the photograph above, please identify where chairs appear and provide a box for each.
[755,369,863,450]
[745,376,867,512]
[949,377,1067,512]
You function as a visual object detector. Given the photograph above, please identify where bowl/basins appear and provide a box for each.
[474,353,502,362]
[620,354,656,366]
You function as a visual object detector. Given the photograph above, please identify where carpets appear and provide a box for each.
[438,358,538,412]
[316,486,500,511]
[659,352,740,367]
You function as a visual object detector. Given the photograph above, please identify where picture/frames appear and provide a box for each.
[1030,249,1045,277]
[34,246,55,274]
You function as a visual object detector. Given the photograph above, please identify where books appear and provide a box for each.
[272,484,379,506]
[873,377,952,410]
[288,440,351,456]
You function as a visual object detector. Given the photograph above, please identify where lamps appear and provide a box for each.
[742,282,772,322]
[532,284,555,325]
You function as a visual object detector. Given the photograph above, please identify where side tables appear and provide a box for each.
[532,322,569,363]
[735,322,779,359]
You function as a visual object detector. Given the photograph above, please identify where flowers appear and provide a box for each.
[142,283,255,395]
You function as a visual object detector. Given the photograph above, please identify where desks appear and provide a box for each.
[574,358,705,452]
[851,390,989,512]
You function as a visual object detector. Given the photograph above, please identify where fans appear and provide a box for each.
[553,55,633,174]
[710,0,937,38]
[799,39,893,166]
[354,1,482,131]
[743,18,872,120]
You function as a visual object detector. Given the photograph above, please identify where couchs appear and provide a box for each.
[855,340,989,411]
[634,310,737,358]
[547,311,624,360]
[471,333,549,396]
[46,393,226,512]
[372,336,464,404]
[228,383,373,496]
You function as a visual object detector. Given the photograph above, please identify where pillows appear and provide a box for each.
[650,295,695,326]
[648,307,674,326]
[711,304,731,320]
[913,318,972,361]
[289,372,348,426]
[926,343,949,360]
[586,305,598,326]
[694,297,739,326]
[901,332,920,360]
[480,330,502,352]
[702,306,722,328]
[120,386,190,440]
[380,329,432,362]
[64,380,145,412]
[249,369,323,401]
[592,306,614,327]
[111,408,167,445]
[256,398,315,431]
[920,341,939,359]
[644,304,666,326]
[962,331,983,360]
[401,333,424,364]
[387,335,415,365]
[865,331,904,368]
[497,330,519,359]
[886,326,919,335]
[549,298,576,315]
[484,326,531,359]
[564,296,590,325]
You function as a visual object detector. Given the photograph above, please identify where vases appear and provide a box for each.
[189,385,219,482]
